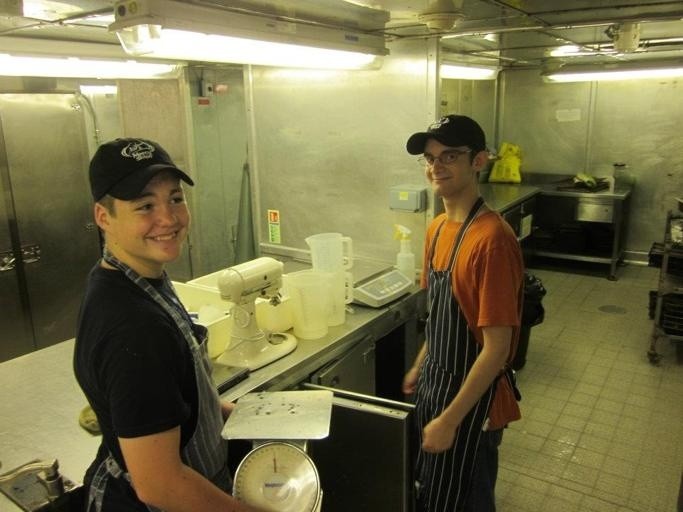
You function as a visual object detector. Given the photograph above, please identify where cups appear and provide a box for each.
[287,231,356,341]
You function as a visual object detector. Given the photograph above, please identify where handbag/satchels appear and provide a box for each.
[488,142,522,183]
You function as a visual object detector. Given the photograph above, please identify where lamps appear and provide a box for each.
[1,36,188,80]
[440,52,504,81]
[108,0,390,72]
[538,60,683,85]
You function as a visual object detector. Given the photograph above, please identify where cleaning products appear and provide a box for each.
[392,223,415,288]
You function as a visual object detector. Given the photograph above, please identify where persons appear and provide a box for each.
[73,139,253,512]
[402,114,525,511]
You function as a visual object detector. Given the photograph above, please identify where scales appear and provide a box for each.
[220,390,334,512]
[344,258,414,307]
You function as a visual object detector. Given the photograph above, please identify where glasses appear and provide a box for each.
[417,149,471,166]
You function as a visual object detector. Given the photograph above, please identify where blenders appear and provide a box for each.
[213,256,299,373]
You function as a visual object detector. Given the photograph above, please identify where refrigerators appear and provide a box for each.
[0,92,102,364]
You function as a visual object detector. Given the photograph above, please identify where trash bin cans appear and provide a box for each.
[512,273,546,370]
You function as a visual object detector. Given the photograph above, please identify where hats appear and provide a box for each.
[89,137,195,201]
[406,114,486,155]
